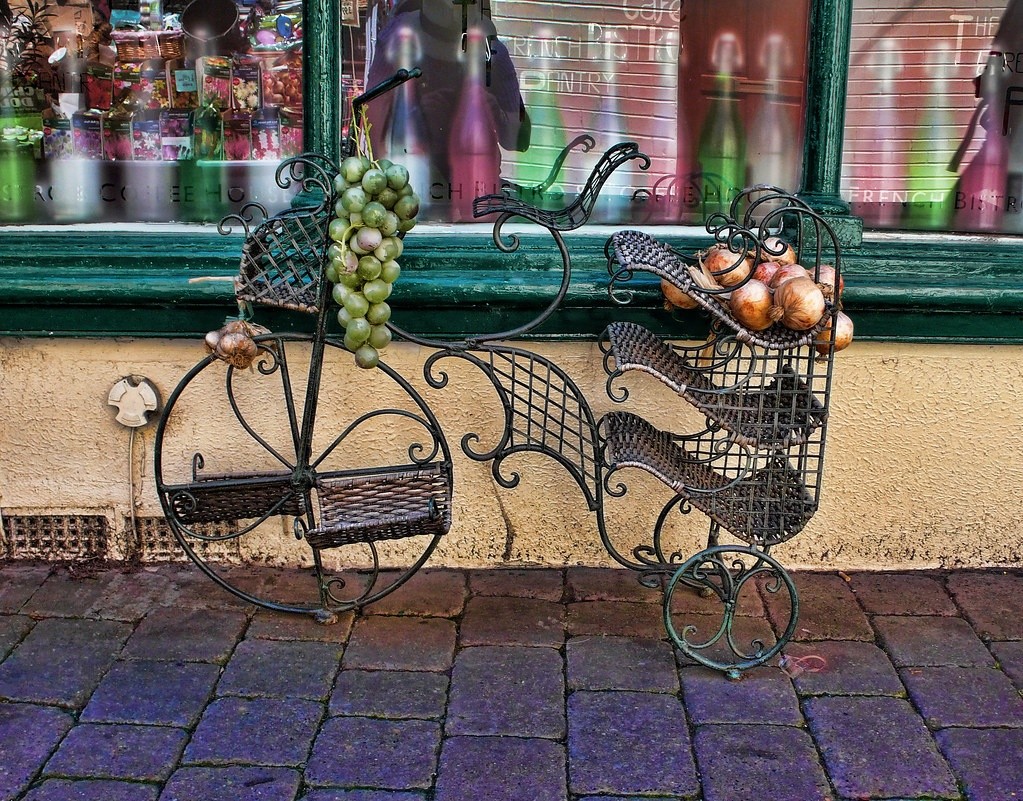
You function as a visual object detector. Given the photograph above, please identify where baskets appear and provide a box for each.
[111,26,187,61]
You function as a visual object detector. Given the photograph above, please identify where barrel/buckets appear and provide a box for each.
[180,0,239,66]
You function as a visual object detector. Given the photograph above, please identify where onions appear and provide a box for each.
[205,321,275,369]
[661,237,854,365]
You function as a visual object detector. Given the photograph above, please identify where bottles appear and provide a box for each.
[852,39,903,229]
[578,29,633,224]
[907,43,960,230]
[643,33,690,224]
[518,29,568,224]
[957,45,1010,232]
[751,34,796,228]
[383,26,435,219]
[697,35,747,224]
[450,27,499,222]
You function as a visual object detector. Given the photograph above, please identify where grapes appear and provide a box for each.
[328,156,421,369]
